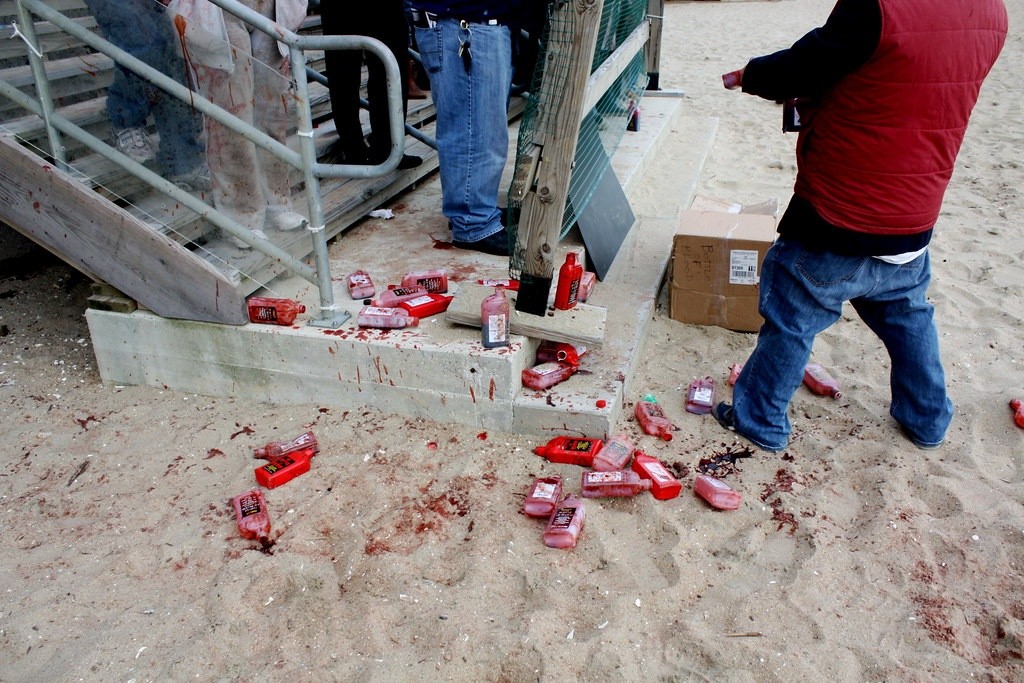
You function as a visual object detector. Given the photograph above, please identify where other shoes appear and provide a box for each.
[404,60,428,99]
[594,114,610,130]
[448,206,523,256]
[598,101,629,117]
[333,142,423,169]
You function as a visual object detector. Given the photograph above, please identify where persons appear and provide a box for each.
[407,0,533,257]
[309,0,428,171]
[699,0,1008,455]
[164,0,314,247]
[85,1,218,189]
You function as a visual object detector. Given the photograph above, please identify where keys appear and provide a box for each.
[455,40,475,73]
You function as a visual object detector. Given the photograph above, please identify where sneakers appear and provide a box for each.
[717,400,788,450]
[223,224,268,251]
[111,123,158,168]
[164,162,216,193]
[271,210,310,232]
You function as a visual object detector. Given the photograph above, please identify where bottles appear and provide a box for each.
[244,294,308,325]
[356,304,420,330]
[534,434,683,502]
[803,362,842,405]
[635,397,673,442]
[541,492,584,547]
[693,473,742,513]
[227,490,276,551]
[249,432,318,484]
[522,339,589,388]
[348,266,374,297]
[554,251,595,310]
[524,474,565,518]
[686,376,718,415]
[728,365,742,380]
[482,287,511,349]
[364,269,455,319]
[1007,399,1024,428]
[475,279,520,291]
